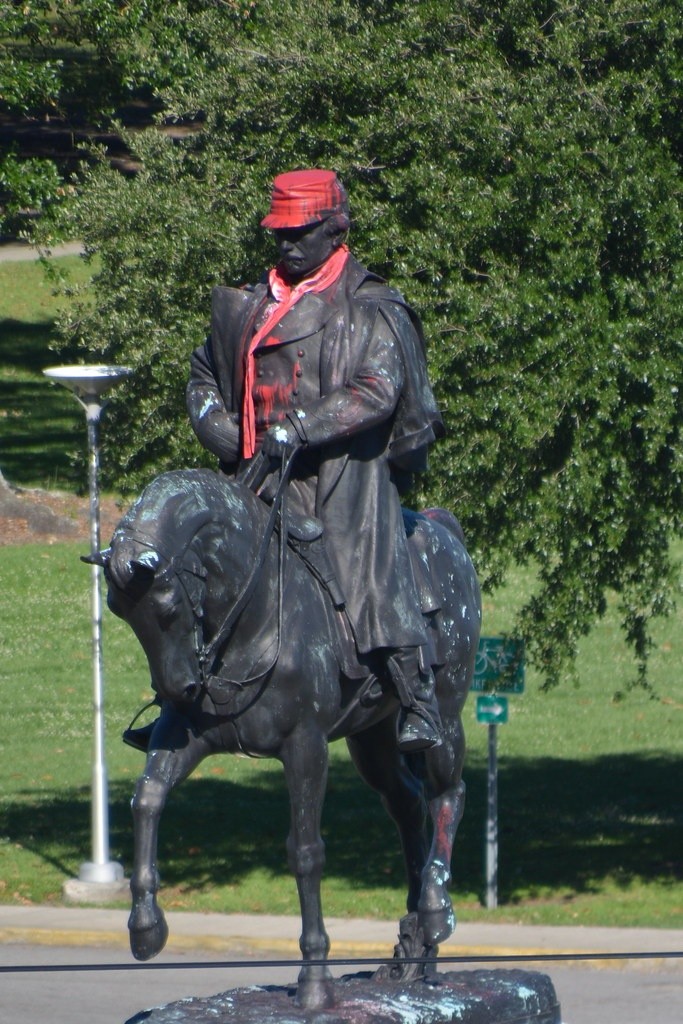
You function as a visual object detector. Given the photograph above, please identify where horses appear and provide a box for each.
[77,466,486,1015]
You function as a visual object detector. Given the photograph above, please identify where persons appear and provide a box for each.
[186,170,447,751]
